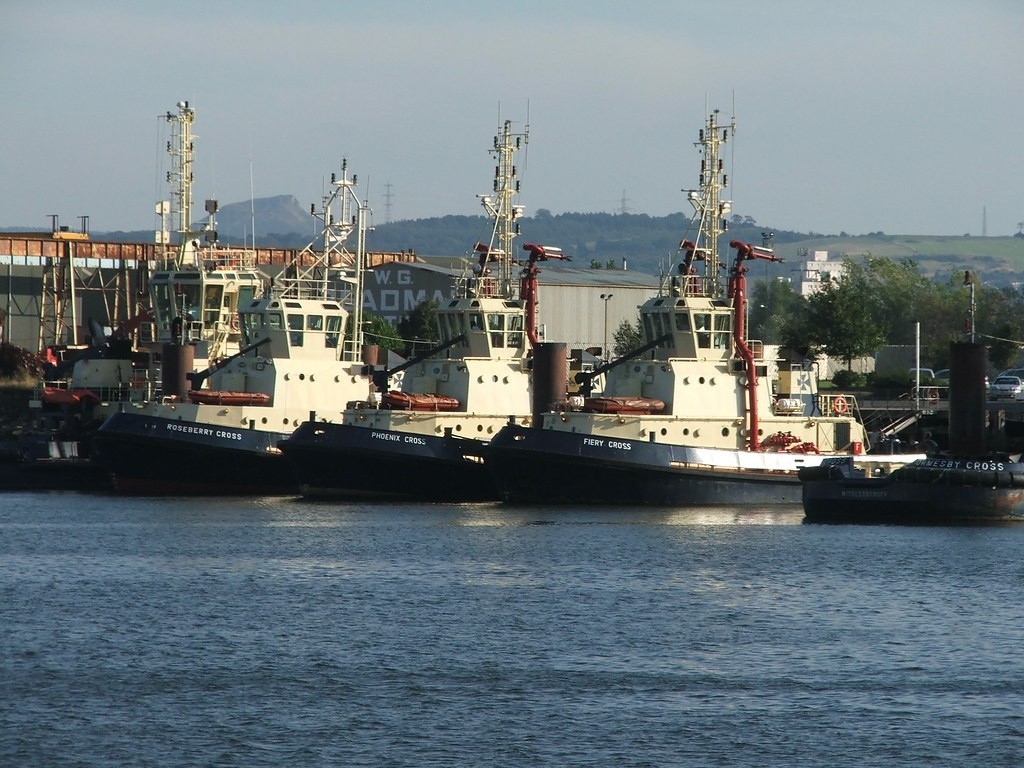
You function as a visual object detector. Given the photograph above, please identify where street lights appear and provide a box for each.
[600,291,610,395]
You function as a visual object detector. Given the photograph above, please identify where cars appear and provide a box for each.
[904,364,935,391]
[932,368,991,398]
[988,377,1024,402]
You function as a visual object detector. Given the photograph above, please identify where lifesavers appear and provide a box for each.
[232,316,240,331]
[834,398,847,413]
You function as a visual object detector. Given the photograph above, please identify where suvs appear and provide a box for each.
[992,369,1024,387]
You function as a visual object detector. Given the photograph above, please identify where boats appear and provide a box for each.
[0,91,931,510]
[796,269,1024,524]
